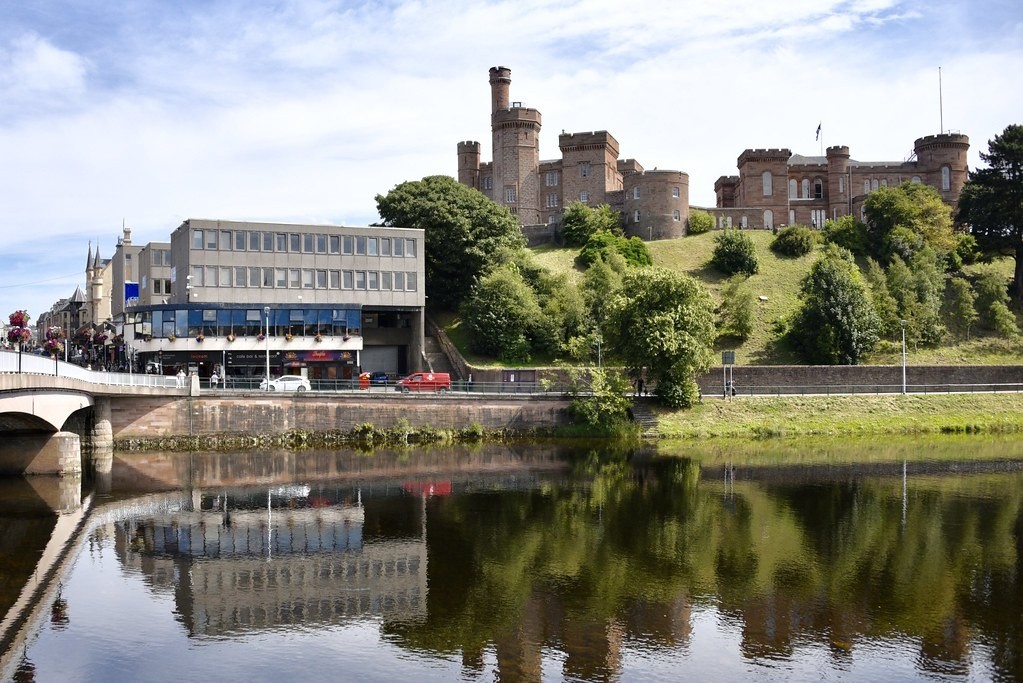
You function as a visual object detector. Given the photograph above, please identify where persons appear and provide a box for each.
[102,366,106,371]
[632,376,644,395]
[467,374,474,391]
[86,364,91,371]
[212,371,218,390]
[727,379,736,395]
[52,353,57,360]
[177,370,186,388]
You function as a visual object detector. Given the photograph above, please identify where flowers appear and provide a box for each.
[286,333,292,341]
[7,310,31,343]
[83,326,124,346]
[168,334,175,342]
[195,332,205,343]
[256,332,265,341]
[144,335,152,344]
[314,334,322,341]
[226,333,235,342]
[42,323,66,354]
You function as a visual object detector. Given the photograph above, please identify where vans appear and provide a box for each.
[394,372,450,394]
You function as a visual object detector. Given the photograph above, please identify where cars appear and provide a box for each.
[401,482,453,499]
[369,371,388,384]
[259,375,311,393]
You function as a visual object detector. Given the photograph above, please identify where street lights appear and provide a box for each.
[264,307,271,392]
